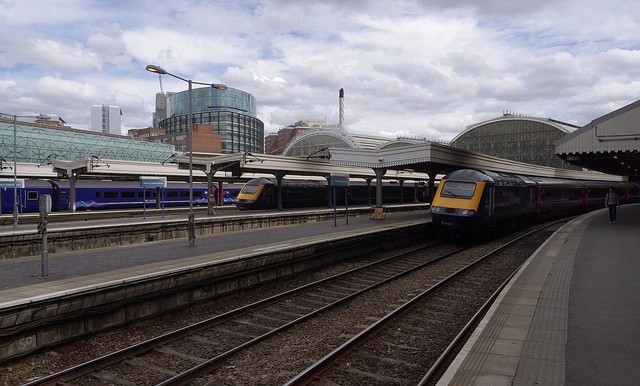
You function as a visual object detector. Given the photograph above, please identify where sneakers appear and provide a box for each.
[613,221,617,224]
[608,219,612,224]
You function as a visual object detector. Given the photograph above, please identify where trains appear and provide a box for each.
[429,169,640,237]
[0,177,246,213]
[235,177,442,211]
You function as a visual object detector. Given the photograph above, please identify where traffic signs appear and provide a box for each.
[37,194,52,277]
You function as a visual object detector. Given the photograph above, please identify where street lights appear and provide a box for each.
[146,65,228,248]
[0,113,51,230]
